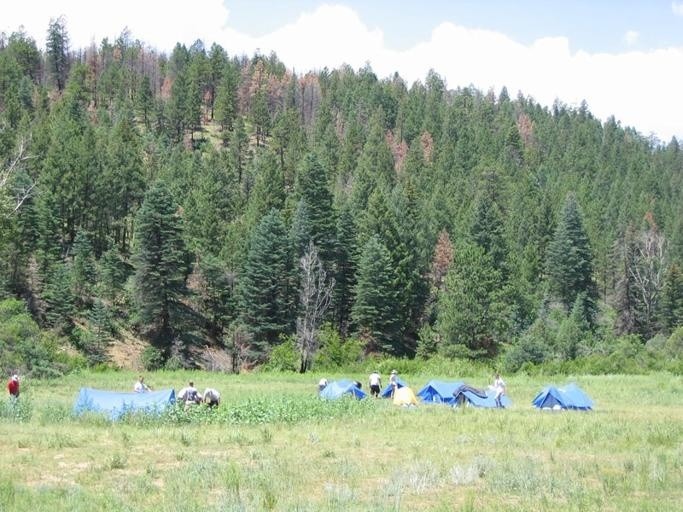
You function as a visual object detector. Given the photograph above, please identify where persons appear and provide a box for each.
[8,376,19,398]
[133,376,150,392]
[319,377,328,391]
[493,374,506,408]
[369,372,382,398]
[183,381,220,408]
[390,370,398,398]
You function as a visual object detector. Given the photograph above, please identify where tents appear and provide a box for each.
[73,389,175,415]
[319,377,593,412]
